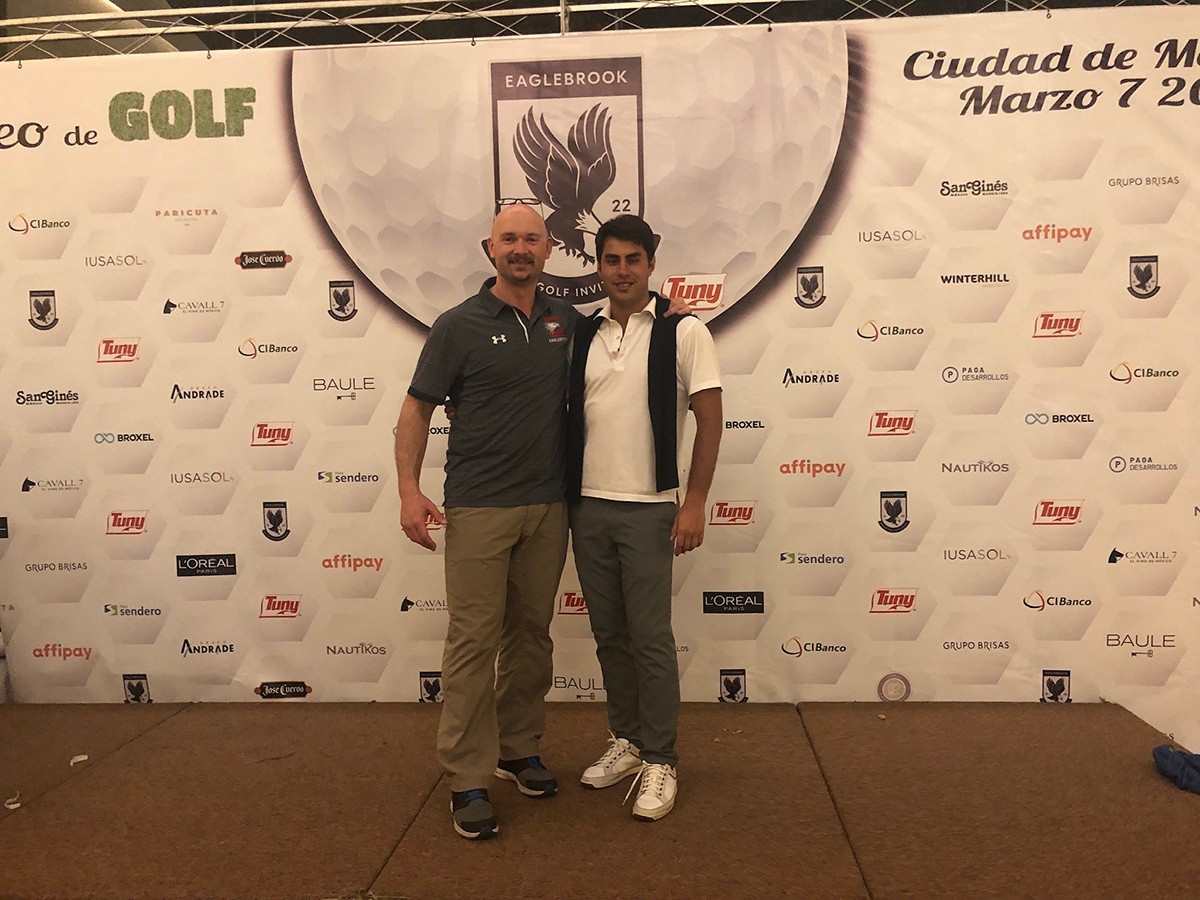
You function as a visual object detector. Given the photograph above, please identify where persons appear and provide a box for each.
[444,213,724,820]
[396,205,693,839]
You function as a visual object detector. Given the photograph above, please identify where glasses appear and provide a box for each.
[493,198,543,220]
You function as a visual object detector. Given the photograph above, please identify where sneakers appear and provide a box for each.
[495,757,558,798]
[580,729,642,789]
[450,790,499,837]
[623,760,678,821]
